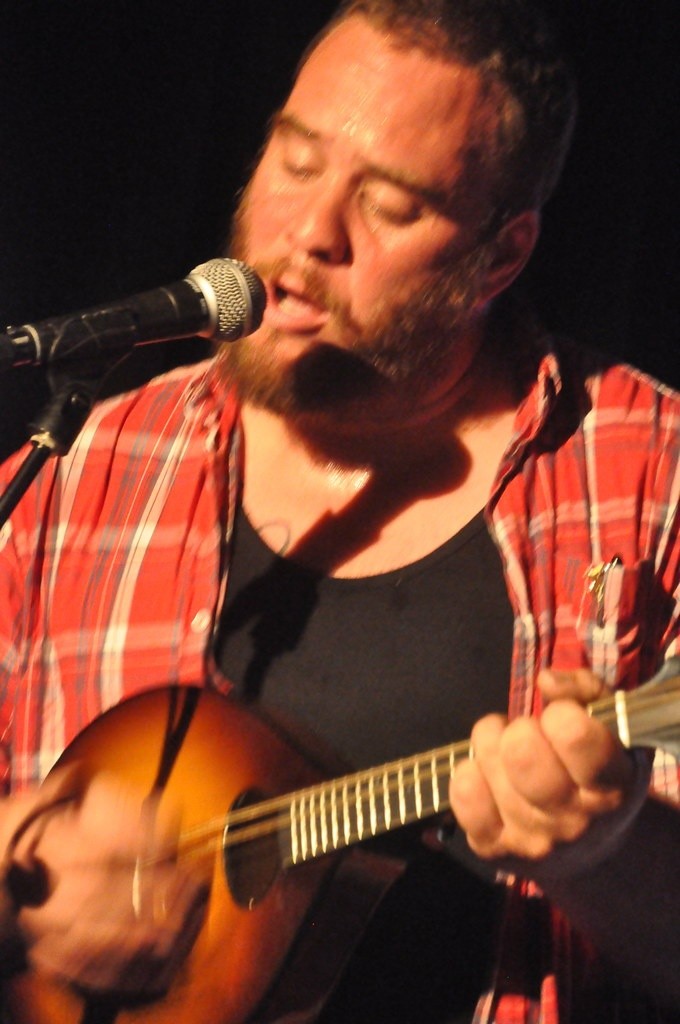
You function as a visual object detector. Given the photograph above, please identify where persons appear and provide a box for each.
[0,0,680,1024]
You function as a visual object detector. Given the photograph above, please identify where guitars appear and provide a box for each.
[0,652,680,1024]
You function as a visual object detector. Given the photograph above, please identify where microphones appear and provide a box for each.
[0,258,268,375]
